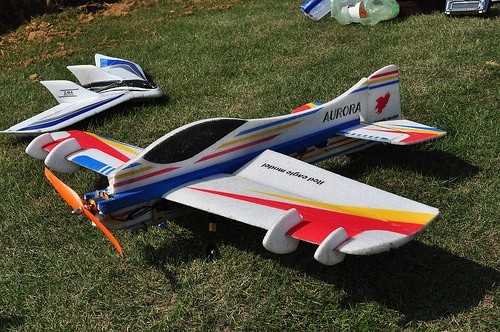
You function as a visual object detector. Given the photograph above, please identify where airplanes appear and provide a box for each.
[2,53,163,136]
[23,65,446,265]
